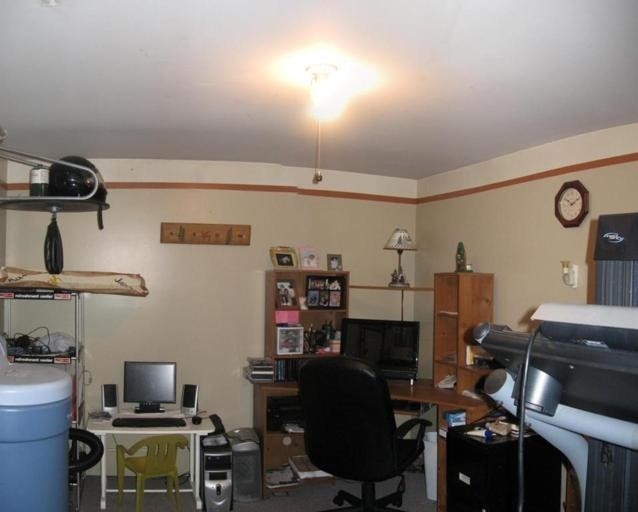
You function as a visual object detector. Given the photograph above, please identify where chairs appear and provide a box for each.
[114,432,191,510]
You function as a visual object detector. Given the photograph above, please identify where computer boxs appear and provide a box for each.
[200,435,234,512]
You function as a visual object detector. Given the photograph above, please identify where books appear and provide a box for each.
[283,422,305,435]
[240,355,305,386]
[464,341,505,371]
[496,417,537,440]
[263,463,300,490]
[287,453,334,481]
[438,309,458,316]
[438,408,466,439]
[437,373,456,389]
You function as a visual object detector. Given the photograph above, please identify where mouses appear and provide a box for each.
[192,416,203,425]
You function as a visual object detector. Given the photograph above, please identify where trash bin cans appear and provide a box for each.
[423,431,437,501]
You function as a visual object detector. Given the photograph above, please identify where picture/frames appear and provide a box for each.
[326,254,341,268]
[269,243,295,267]
[275,276,298,308]
[298,244,321,268]
[277,325,305,354]
[304,274,339,306]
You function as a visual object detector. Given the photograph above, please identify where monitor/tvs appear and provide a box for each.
[340,318,421,382]
[124,360,177,413]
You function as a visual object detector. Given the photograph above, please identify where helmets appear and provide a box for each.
[49,156,106,202]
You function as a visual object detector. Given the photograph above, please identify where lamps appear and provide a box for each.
[381,224,414,285]
[278,44,360,186]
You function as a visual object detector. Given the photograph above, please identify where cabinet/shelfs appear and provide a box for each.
[431,274,480,390]
[11,280,86,511]
[263,265,340,383]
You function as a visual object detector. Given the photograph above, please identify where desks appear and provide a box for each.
[250,381,502,512]
[88,414,215,511]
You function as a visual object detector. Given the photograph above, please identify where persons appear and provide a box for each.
[276,253,341,307]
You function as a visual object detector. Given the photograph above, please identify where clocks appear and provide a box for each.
[553,177,589,226]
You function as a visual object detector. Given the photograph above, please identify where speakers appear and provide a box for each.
[101,383,119,414]
[181,384,199,418]
